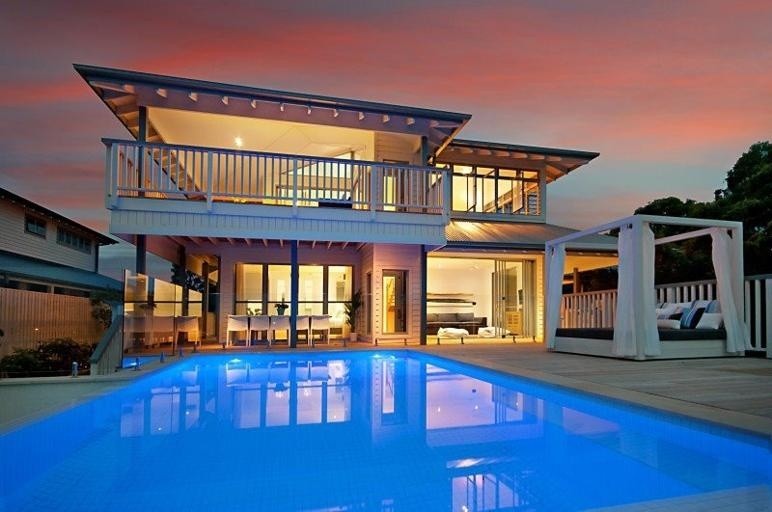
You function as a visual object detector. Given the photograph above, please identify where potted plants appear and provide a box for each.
[344,288,363,342]
[274,304,288,315]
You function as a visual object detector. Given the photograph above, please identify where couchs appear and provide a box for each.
[426,317,487,335]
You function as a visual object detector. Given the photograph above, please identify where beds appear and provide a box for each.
[555,328,745,361]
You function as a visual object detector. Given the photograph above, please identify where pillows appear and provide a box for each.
[656,303,663,309]
[674,306,705,328]
[457,312,474,321]
[693,300,721,313]
[656,312,684,330]
[696,312,722,329]
[438,313,457,321]
[661,301,693,309]
[426,313,438,322]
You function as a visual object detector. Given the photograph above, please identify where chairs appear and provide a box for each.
[296,315,310,346]
[310,315,330,346]
[269,315,291,347]
[248,315,270,346]
[123,316,202,352]
[225,314,249,348]
